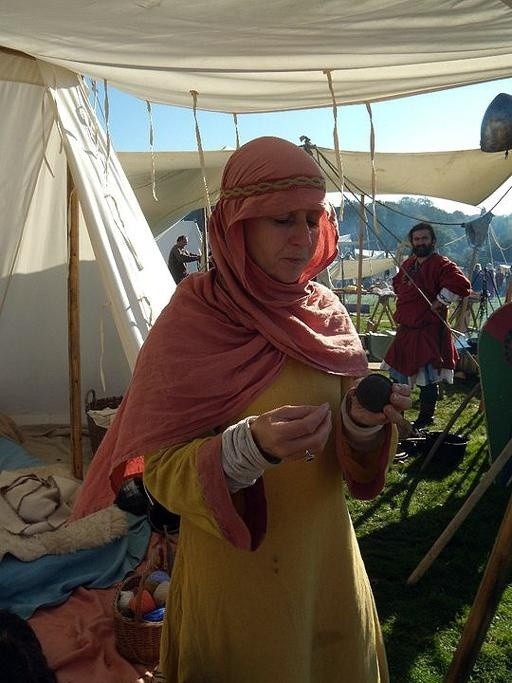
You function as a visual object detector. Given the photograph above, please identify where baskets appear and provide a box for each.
[84,389,123,457]
[112,560,167,666]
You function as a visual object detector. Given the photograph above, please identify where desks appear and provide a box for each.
[331,289,398,332]
[448,291,482,329]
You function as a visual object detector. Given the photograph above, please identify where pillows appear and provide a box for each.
[0,413,26,445]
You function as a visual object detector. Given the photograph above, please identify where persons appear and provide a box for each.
[379,222,474,427]
[468,261,512,301]
[127,136,416,682]
[167,233,201,286]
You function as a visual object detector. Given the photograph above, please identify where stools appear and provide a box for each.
[358,332,398,362]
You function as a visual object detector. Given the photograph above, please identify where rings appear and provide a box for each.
[304,448,318,463]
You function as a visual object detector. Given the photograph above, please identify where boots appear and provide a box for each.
[411,384,439,429]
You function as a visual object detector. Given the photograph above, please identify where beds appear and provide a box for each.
[0,410,153,683]
